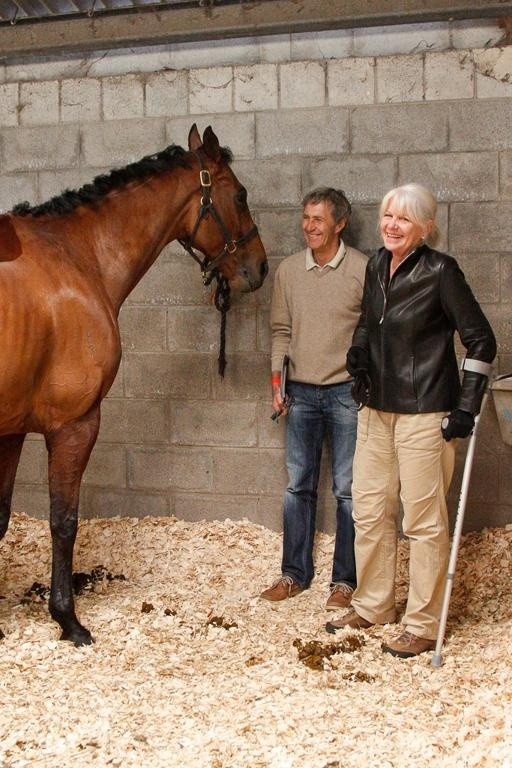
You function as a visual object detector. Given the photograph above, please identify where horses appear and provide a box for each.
[0,123,269,648]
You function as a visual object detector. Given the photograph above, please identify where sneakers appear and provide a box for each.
[324,582,354,610]
[326,609,397,634]
[262,576,307,601]
[385,631,446,657]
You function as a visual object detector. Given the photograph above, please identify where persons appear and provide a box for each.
[326,182,498,658]
[260,186,371,611]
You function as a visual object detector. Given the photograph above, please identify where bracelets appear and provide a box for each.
[271,377,281,386]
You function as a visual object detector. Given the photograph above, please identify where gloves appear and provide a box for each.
[441,409,474,441]
[346,346,369,377]
